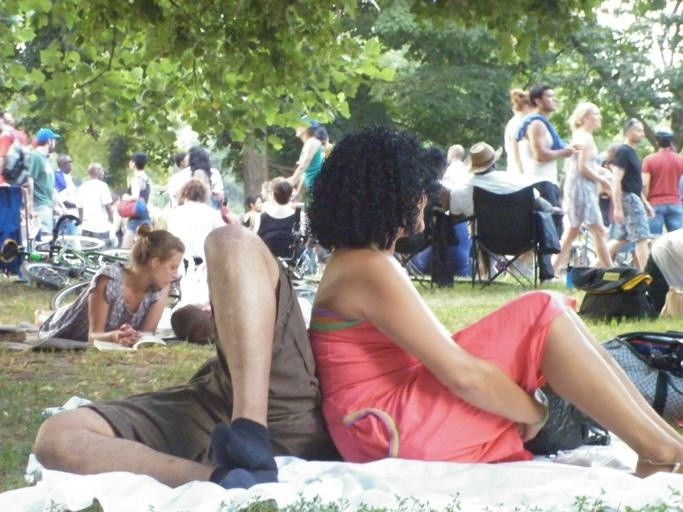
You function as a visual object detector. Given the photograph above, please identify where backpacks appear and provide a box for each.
[0,130,25,183]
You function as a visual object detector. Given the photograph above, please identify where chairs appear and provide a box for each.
[0,185,32,281]
[395,210,438,292]
[255,205,305,281]
[470,181,541,290]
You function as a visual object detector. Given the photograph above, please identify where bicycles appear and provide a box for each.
[0,216,185,319]
[265,231,320,306]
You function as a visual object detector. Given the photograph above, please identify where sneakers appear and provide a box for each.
[540,275,562,285]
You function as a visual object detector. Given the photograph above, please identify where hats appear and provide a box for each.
[466,140,504,174]
[56,154,74,165]
[298,115,319,128]
[35,128,62,142]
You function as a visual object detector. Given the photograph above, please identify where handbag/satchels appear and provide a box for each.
[117,198,147,218]
[524,369,613,456]
[584,328,683,421]
[572,265,658,320]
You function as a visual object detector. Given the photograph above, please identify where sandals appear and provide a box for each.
[630,456,683,479]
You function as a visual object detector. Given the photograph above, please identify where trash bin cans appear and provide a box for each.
[0,184,24,274]
